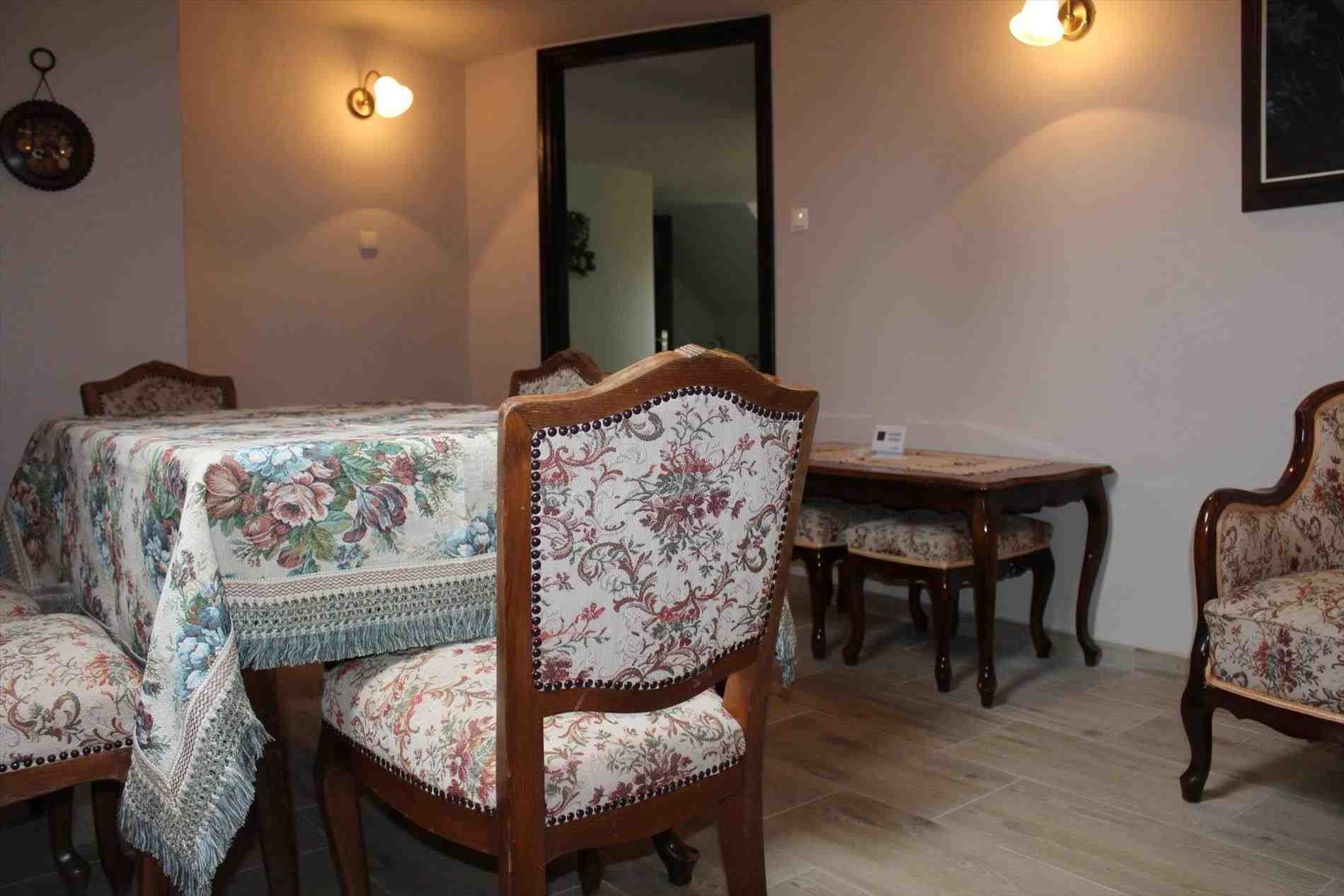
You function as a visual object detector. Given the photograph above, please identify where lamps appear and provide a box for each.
[347,70,414,119]
[1009,0,1096,46]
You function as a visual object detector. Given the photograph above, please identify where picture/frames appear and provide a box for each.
[1241,0,1344,213]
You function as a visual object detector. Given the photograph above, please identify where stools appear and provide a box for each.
[842,515,1056,693]
[771,499,886,662]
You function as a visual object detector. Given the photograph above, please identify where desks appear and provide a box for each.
[0,400,500,896]
[791,441,1117,707]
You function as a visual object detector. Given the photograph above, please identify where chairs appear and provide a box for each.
[0,344,824,896]
[1179,380,1344,802]
